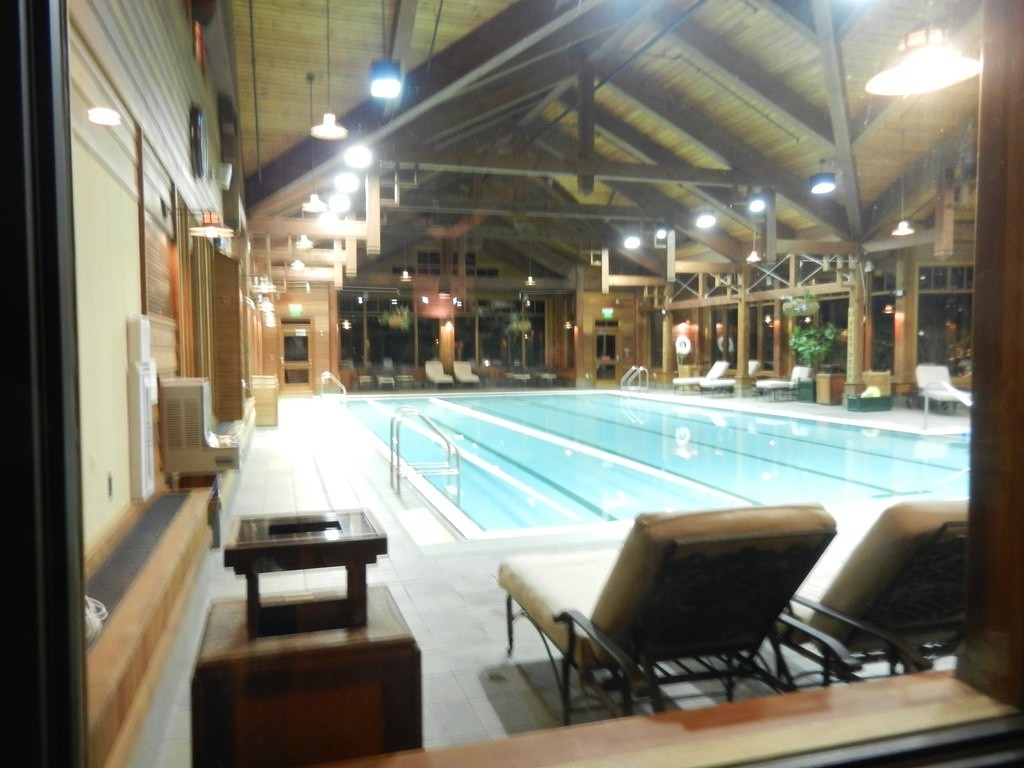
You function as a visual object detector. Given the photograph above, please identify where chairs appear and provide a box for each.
[506,371,530,384]
[672,359,730,396]
[533,371,559,384]
[915,362,973,413]
[698,359,761,397]
[768,488,972,682]
[498,502,841,727]
[452,360,480,386]
[425,359,453,385]
[756,364,813,400]
[377,374,395,389]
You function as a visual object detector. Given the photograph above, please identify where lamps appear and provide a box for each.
[84,0,983,351]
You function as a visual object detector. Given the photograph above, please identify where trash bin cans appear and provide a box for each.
[191,507,423,767]
[338,360,353,390]
[696,361,711,392]
[816,364,846,406]
[250,375,279,427]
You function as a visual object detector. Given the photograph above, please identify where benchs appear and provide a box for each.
[83,485,215,768]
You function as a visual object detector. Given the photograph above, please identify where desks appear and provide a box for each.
[191,583,422,768]
[224,507,387,643]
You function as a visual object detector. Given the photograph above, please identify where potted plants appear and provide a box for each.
[787,322,841,400]
